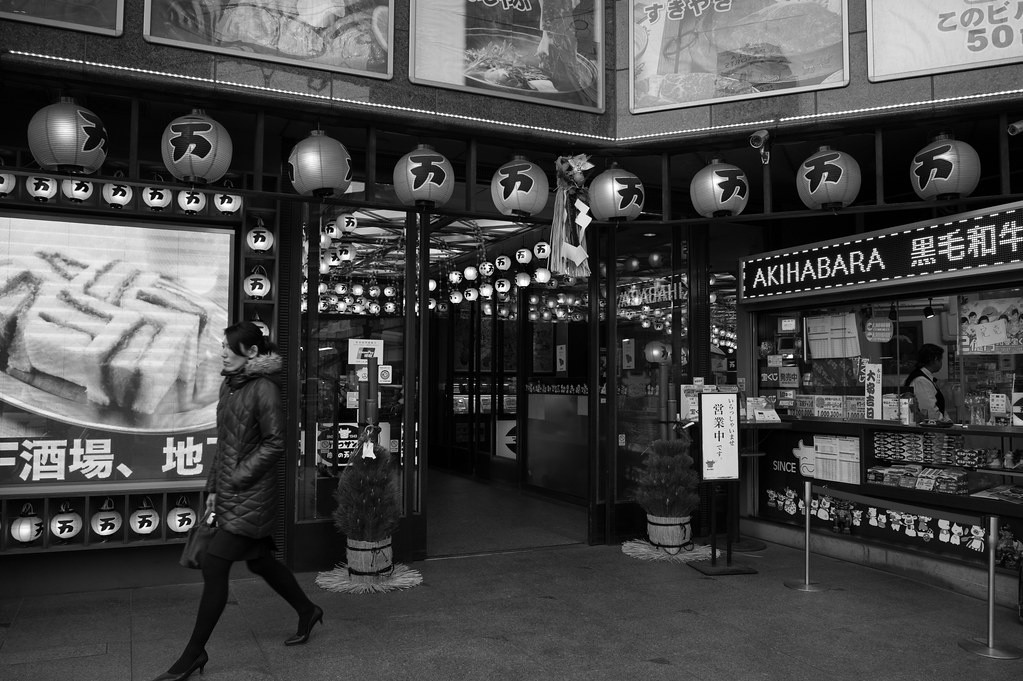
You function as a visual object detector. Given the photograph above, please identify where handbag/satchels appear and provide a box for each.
[178,508,218,569]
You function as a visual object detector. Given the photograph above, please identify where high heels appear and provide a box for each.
[286,605,324,645]
[153,648,208,680]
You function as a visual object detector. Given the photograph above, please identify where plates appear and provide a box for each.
[0,283,228,436]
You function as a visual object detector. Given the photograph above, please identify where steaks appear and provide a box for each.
[688,2,844,83]
[534,0,580,90]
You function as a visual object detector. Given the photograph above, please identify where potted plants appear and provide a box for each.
[635,439,699,554]
[333,439,401,582]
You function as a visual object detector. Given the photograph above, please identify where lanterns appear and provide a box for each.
[795,146,861,208]
[300,213,742,353]
[161,110,234,186]
[286,130,355,198]
[394,145,455,210]
[29,95,111,174]
[10,497,216,544]
[244,223,275,338]
[586,168,646,221]
[490,154,550,218]
[909,133,982,200]
[690,158,748,218]
[0,172,244,217]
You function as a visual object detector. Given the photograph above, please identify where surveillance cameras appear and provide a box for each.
[749,130,769,164]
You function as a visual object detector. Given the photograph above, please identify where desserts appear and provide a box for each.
[0,250,227,416]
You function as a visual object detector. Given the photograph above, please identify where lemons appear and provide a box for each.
[371,6,388,52]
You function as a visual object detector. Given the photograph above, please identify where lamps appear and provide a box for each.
[690,159,749,218]
[161,107,232,185]
[129,506,160,536]
[167,506,196,534]
[490,156,549,216]
[288,130,353,198]
[796,146,862,210]
[103,183,133,208]
[910,135,981,201]
[142,187,173,211]
[61,179,93,203]
[924,307,935,319]
[91,508,122,535]
[177,191,206,215]
[301,213,738,361]
[11,514,43,545]
[393,143,455,208]
[50,509,83,539]
[0,173,16,198]
[28,97,109,175]
[214,194,242,215]
[26,176,58,202]
[588,163,644,222]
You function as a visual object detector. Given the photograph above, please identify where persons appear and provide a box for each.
[151,321,323,681]
[899,342,955,426]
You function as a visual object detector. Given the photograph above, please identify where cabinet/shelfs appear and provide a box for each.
[739,412,1023,577]
